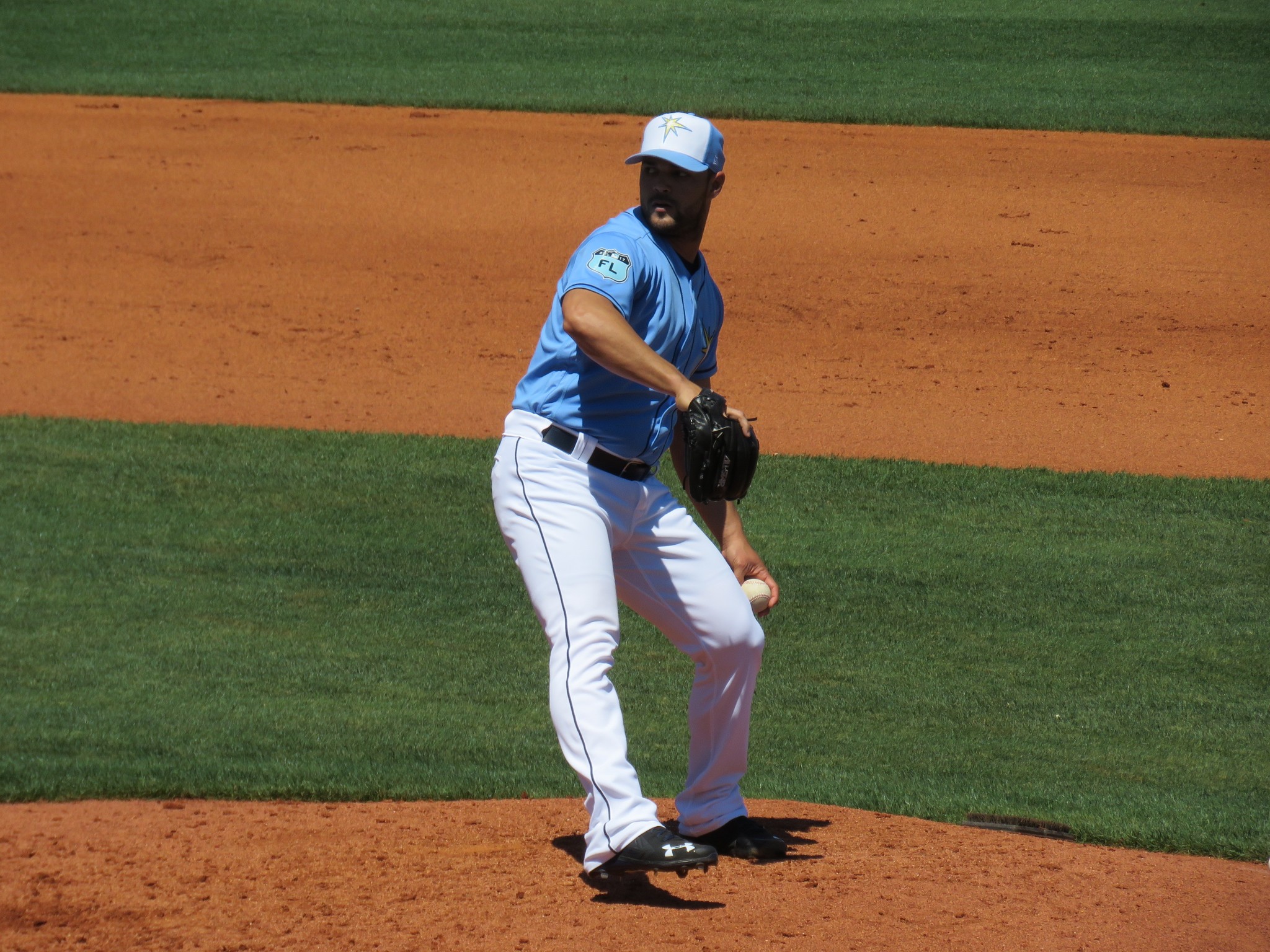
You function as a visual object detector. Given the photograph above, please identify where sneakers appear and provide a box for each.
[679,816,788,860]
[590,826,719,885]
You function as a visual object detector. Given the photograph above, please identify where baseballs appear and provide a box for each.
[741,578,771,613]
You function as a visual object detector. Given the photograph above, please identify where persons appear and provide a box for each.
[489,111,788,888]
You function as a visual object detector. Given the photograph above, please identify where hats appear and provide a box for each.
[626,112,726,179]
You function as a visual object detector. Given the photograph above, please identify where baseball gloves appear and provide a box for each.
[680,386,760,504]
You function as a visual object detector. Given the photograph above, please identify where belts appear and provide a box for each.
[542,423,653,482]
